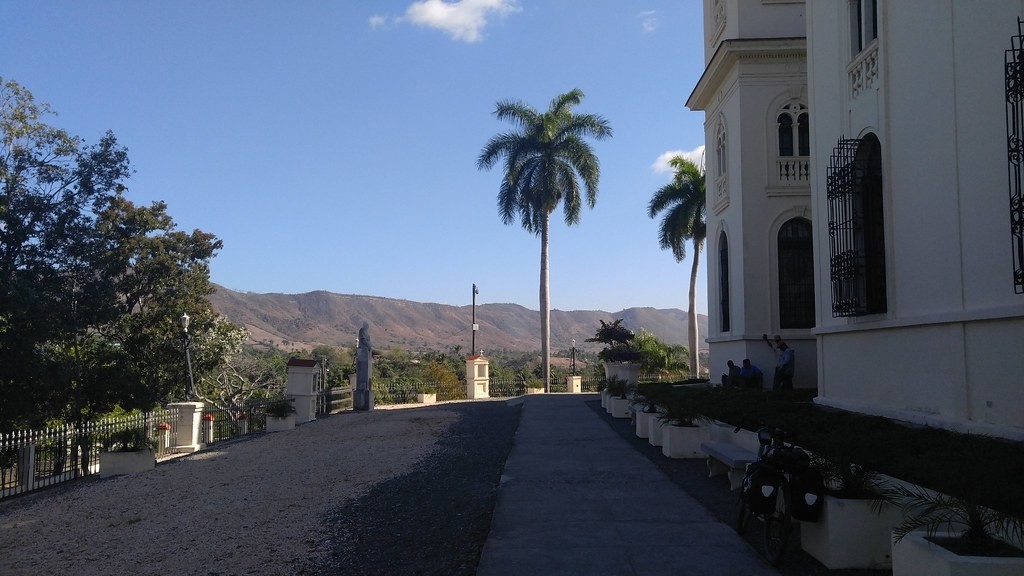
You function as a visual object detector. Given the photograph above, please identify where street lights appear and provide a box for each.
[180,311,191,401]
[572,339,575,375]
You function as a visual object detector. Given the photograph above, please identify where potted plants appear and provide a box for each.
[527,379,545,395]
[796,423,909,571]
[416,385,437,403]
[98,426,160,479]
[584,318,644,388]
[262,399,299,432]
[880,430,1024,576]
[595,376,725,459]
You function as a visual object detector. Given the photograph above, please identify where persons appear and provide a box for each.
[776,341,795,396]
[762,333,783,393]
[358,321,372,349]
[739,359,761,377]
[727,360,741,376]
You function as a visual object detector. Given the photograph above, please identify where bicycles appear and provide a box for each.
[734,419,798,566]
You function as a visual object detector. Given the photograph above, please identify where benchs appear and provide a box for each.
[701,440,761,495]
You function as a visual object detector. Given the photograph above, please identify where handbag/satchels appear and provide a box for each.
[741,462,774,505]
[776,444,823,522]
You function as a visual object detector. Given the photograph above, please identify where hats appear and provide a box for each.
[774,335,781,341]
[776,341,784,348]
